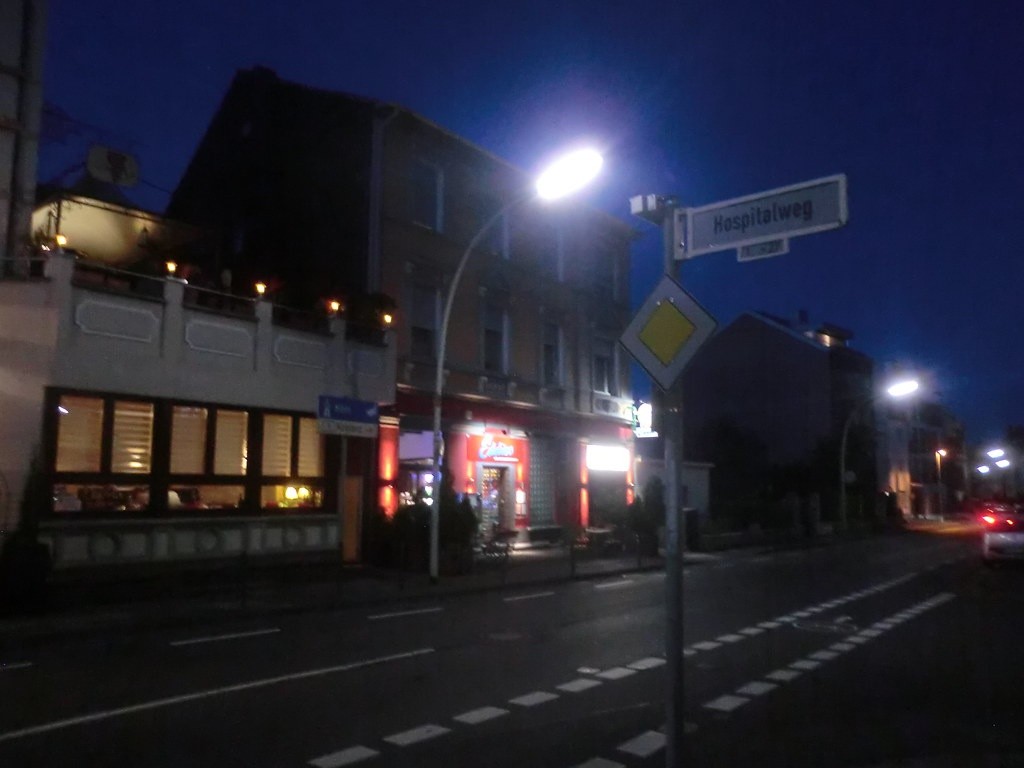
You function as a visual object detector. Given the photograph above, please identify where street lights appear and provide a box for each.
[838,380,919,542]
[429,148,605,581]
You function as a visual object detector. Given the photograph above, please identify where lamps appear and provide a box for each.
[252,278,268,297]
[54,231,69,256]
[380,309,394,331]
[329,296,345,315]
[163,260,178,276]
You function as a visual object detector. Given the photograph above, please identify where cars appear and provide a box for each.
[975,505,1020,532]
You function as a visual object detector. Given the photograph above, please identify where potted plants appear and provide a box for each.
[395,493,481,574]
